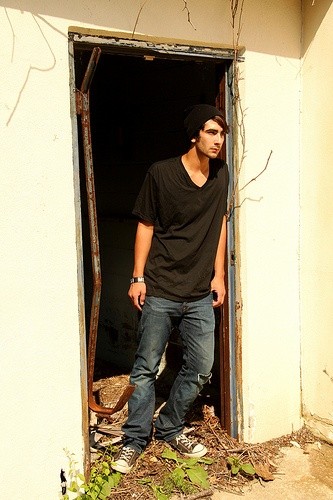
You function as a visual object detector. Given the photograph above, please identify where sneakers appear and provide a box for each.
[110,445,139,474]
[170,435,208,459]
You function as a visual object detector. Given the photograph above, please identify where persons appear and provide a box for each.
[110,104,230,474]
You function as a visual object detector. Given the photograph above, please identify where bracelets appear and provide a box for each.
[129,277,144,284]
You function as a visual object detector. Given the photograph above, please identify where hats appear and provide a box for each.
[184,104,224,138]
